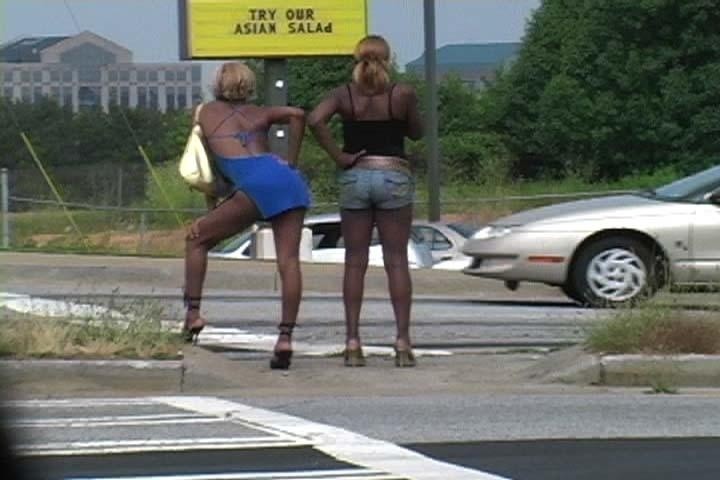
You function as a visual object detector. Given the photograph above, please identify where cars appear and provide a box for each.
[206,213,475,272]
[456,165,720,309]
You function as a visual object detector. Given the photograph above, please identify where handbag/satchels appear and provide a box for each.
[178,120,218,199]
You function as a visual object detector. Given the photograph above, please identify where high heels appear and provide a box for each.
[392,335,418,368]
[269,322,294,370]
[180,298,207,348]
[344,335,368,367]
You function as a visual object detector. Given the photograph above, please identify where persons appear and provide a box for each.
[305,36,426,369]
[171,61,312,369]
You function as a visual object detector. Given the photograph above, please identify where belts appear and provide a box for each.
[349,155,412,177]
